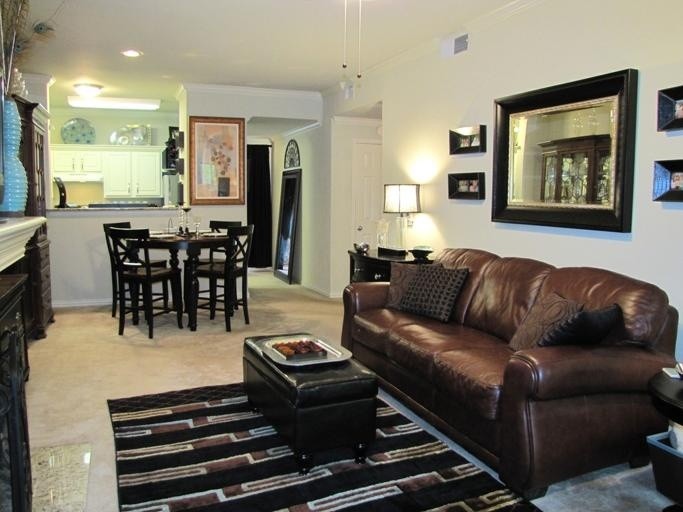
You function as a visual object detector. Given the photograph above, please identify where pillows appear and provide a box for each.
[384,248,669,353]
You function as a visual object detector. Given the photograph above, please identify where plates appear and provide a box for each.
[148,234,176,239]
[60,118,96,145]
[202,232,227,236]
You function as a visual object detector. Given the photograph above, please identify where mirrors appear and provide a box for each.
[273,169,302,285]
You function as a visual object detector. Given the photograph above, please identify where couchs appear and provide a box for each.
[341,249,679,500]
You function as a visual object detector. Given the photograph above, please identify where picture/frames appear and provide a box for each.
[652,159,683,202]
[448,172,485,200]
[491,68,638,233]
[448,125,486,155]
[190,115,246,205]
[657,84,683,133]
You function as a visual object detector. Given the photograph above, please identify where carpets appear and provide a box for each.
[107,383,544,512]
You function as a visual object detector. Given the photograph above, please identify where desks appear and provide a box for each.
[348,249,435,284]
[649,367,683,426]
[0,274,29,383]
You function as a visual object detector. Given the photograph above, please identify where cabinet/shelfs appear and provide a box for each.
[50,144,169,200]
[538,134,612,204]
[11,94,56,340]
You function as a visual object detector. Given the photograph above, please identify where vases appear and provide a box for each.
[0,98,28,217]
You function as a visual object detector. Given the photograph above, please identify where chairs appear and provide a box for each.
[104,221,255,339]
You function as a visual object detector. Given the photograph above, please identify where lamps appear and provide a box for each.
[383,184,421,249]
[74,83,103,98]
[67,96,162,112]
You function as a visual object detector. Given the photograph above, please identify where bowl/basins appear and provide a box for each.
[408,250,434,260]
